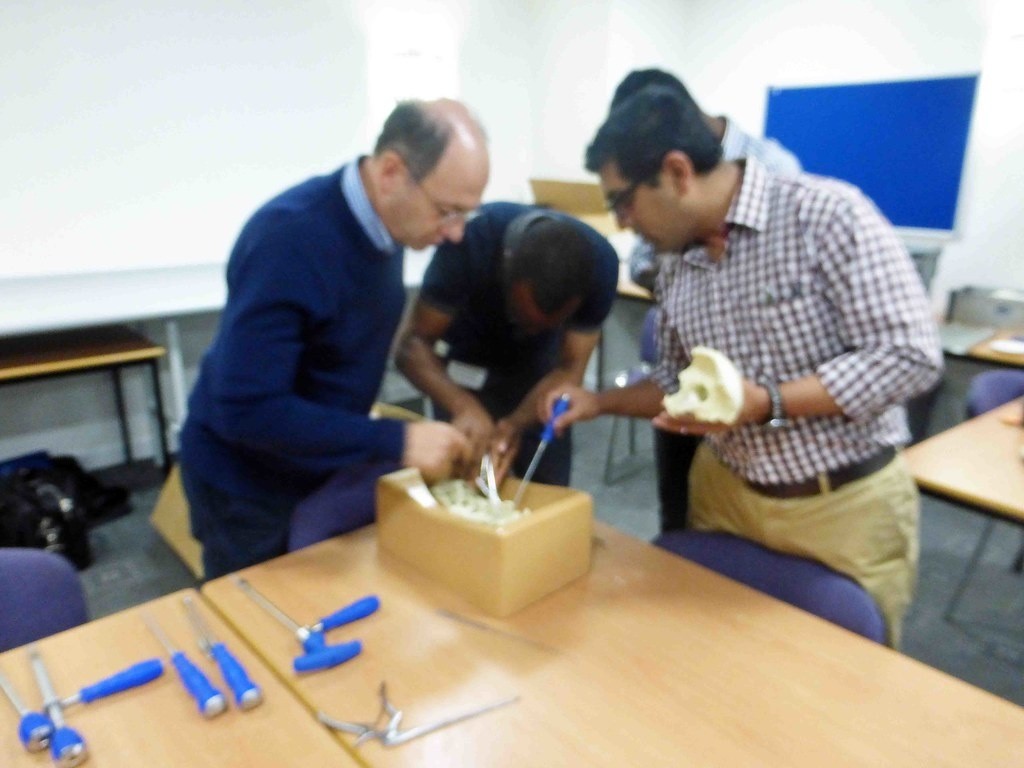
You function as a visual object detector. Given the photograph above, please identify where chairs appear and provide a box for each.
[0,548,87,653]
[966,367,1024,418]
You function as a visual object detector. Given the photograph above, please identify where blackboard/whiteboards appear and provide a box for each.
[765,70,982,241]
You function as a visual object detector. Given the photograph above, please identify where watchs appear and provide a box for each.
[752,372,794,433]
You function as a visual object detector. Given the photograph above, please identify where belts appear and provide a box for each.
[745,446,898,498]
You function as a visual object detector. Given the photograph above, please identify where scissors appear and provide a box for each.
[455,434,502,508]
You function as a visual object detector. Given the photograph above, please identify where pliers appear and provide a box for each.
[316,679,520,747]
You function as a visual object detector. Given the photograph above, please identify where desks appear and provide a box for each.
[577,211,653,484]
[0,327,172,466]
[0,264,226,427]
[940,320,1024,367]
[903,397,1023,621]
[0,514,1024,768]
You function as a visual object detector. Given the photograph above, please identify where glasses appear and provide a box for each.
[399,149,481,224]
[605,162,664,211]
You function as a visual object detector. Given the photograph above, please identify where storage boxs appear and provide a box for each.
[146,397,423,582]
[373,467,593,618]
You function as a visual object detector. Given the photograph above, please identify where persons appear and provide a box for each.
[393,200,619,489]
[538,70,946,652]
[175,95,489,588]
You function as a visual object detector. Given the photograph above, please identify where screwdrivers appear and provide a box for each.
[182,597,265,710]
[312,596,379,633]
[29,648,85,767]
[0,678,51,749]
[144,612,226,718]
[512,392,572,516]
[61,659,162,713]
[235,575,361,670]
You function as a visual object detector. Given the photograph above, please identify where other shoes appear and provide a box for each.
[46,453,129,528]
